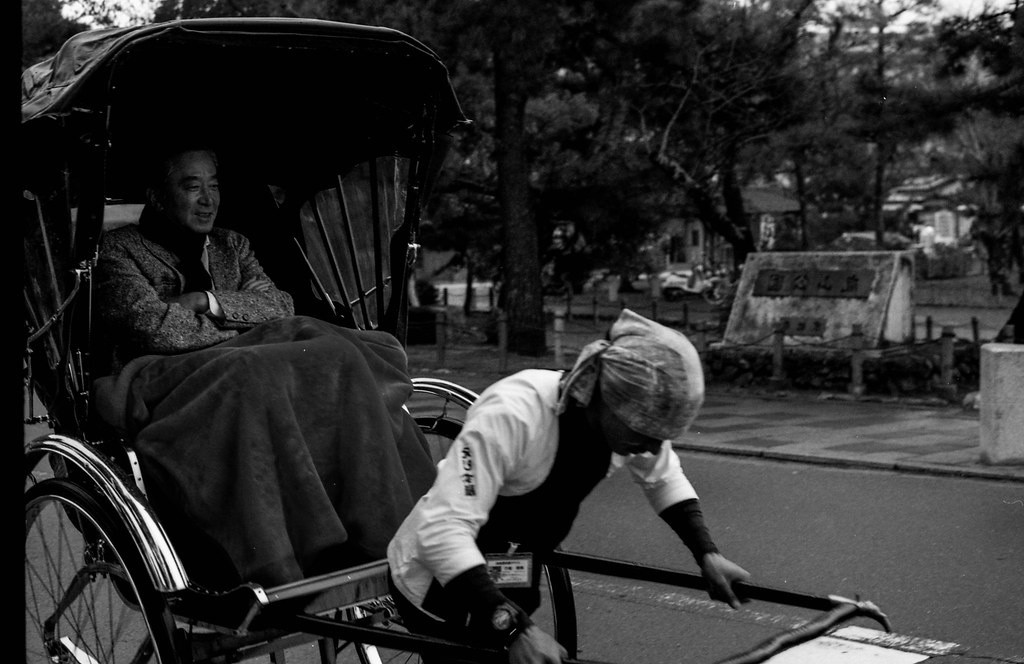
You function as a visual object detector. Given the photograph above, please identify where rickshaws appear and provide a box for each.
[15,14,895,663]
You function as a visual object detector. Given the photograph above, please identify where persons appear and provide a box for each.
[83,145,437,584]
[386,309,756,664]
